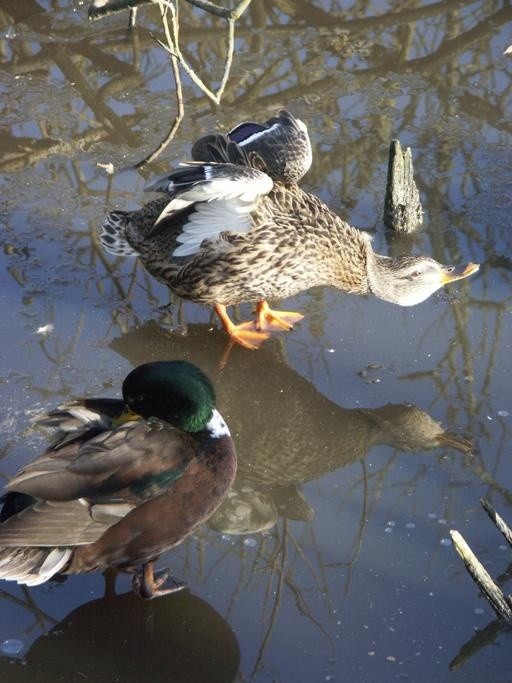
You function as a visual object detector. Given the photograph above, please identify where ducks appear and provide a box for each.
[108,320,476,537]
[97,108,481,353]
[0,358,237,606]
